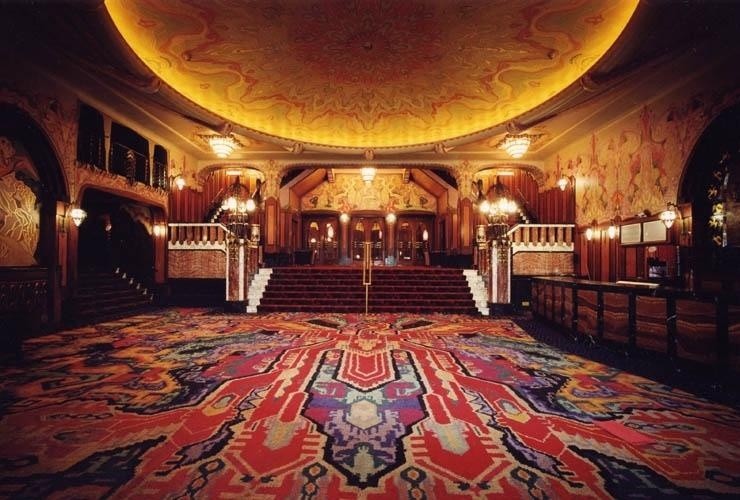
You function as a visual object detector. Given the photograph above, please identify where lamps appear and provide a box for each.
[661,201,682,230]
[65,199,89,229]
[195,130,245,159]
[491,130,548,160]
